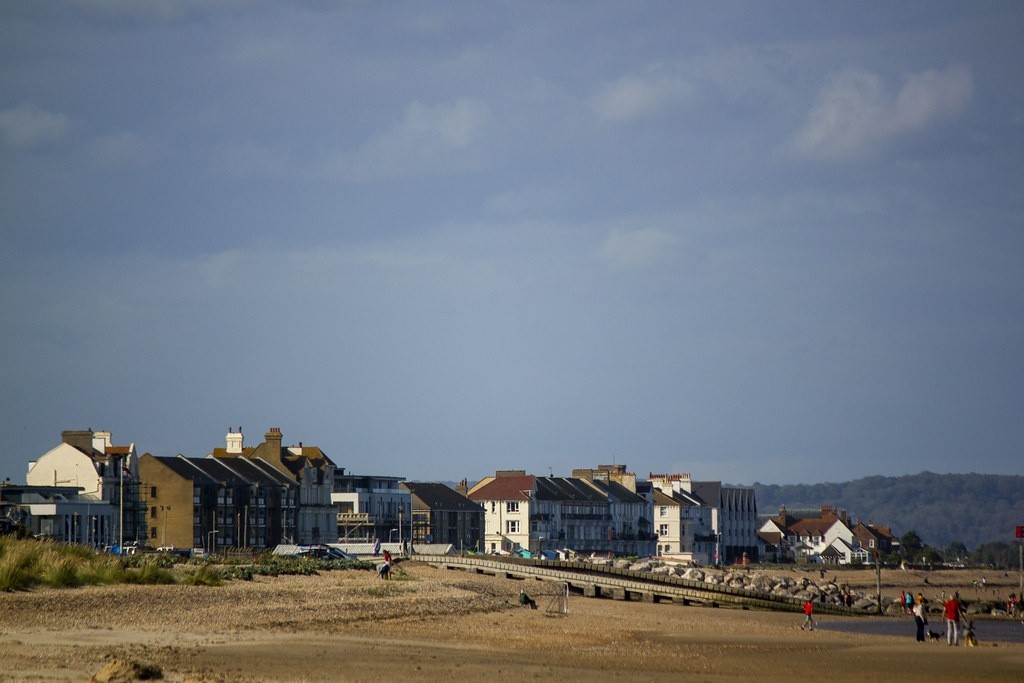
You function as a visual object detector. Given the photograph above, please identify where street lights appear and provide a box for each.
[208,530,219,556]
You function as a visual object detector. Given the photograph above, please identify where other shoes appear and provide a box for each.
[809,628,813,630]
[801,625,804,631]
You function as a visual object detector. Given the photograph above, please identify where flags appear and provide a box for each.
[122,466,131,477]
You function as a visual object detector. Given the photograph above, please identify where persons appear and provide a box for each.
[913,593,926,642]
[941,592,976,647]
[376,549,393,579]
[2,508,20,535]
[403,537,408,553]
[906,592,915,615]
[1006,593,1024,617]
[565,551,569,560]
[801,600,813,630]
[900,590,906,611]
[520,588,537,609]
[373,538,380,556]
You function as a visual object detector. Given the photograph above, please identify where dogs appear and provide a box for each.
[963,622,977,648]
[927,629,944,643]
